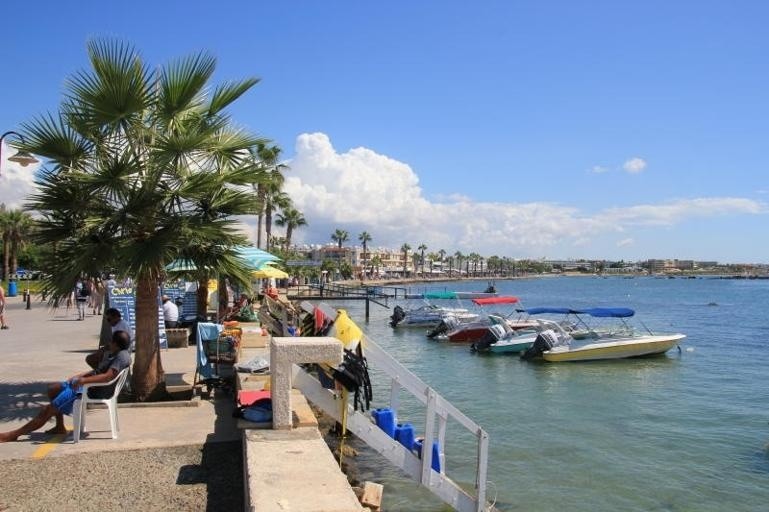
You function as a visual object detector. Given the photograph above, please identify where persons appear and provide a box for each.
[104,273,117,311]
[88,276,96,308]
[173,297,183,328]
[0,285,10,330]
[218,291,248,318]
[0,330,132,444]
[86,307,134,372]
[92,276,106,315]
[159,294,179,328]
[74,277,85,321]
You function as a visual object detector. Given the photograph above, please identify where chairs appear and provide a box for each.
[72,365,129,446]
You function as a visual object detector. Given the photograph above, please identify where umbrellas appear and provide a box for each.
[263,260,279,266]
[198,262,290,300]
[156,240,283,322]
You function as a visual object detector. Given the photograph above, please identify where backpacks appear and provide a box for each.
[231,397,273,423]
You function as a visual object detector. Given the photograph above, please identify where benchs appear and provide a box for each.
[165,324,192,347]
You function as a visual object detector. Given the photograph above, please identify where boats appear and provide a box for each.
[388,290,688,364]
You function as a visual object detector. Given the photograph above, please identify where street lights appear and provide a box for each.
[0,131,40,167]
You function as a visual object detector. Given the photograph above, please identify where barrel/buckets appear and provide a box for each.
[371,406,395,435]
[393,423,416,450]
[413,439,440,474]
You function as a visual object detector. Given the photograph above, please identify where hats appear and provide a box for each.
[162,294,172,301]
